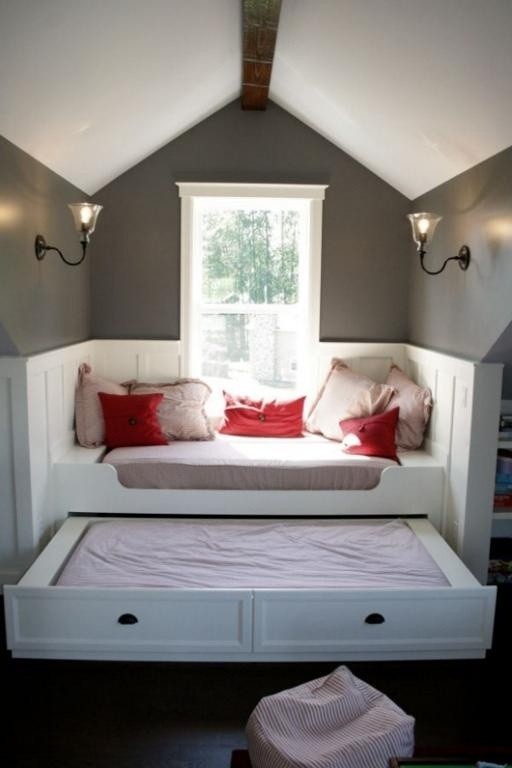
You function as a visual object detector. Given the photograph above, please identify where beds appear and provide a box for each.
[1,399,500,664]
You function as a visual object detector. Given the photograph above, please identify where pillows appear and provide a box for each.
[97,391,169,446]
[301,358,396,441]
[340,406,403,467]
[73,362,129,448]
[382,359,434,450]
[121,378,215,442]
[219,390,305,439]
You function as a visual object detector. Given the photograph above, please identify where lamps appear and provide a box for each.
[407,212,472,275]
[33,201,104,265]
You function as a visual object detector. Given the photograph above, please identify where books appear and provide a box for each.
[497,415,511,441]
[494,456,511,512]
